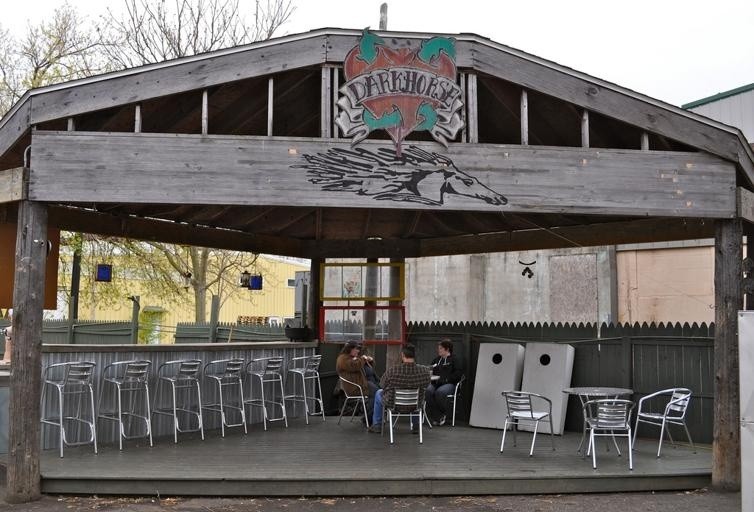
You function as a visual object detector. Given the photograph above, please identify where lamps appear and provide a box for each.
[240,251,263,291]
[94,265,113,282]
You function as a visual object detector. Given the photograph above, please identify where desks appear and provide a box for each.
[393,375,440,429]
[562,387,633,456]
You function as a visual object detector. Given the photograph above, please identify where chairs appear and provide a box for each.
[202,358,247,438]
[582,398,636,471]
[95,360,153,451]
[422,374,465,426]
[336,376,369,429]
[39,360,97,457]
[632,387,696,457]
[500,390,556,458]
[243,356,288,431]
[278,354,325,424]
[382,388,424,444]
[150,359,204,444]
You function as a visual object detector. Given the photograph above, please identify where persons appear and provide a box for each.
[424,339,464,426]
[336,339,380,426]
[369,345,430,433]
